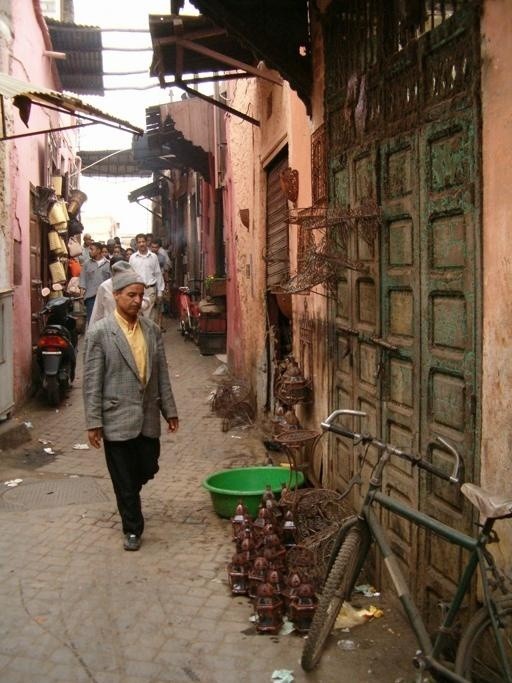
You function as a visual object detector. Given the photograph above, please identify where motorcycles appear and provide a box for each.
[36,283,84,407]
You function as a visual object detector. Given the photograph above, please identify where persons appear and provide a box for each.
[83,260,178,551]
[78,234,172,342]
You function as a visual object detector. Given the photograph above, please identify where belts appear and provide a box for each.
[149,284,156,287]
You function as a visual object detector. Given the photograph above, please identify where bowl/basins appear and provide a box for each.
[202,467,305,517]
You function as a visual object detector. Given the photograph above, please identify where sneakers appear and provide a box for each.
[123,531,141,551]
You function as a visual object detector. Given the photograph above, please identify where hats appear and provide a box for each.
[110,260,148,292]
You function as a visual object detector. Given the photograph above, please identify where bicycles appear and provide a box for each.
[301,409,512,683]
